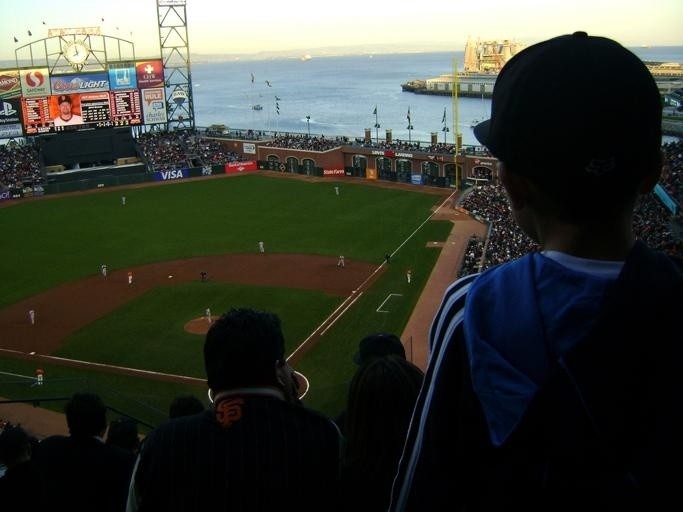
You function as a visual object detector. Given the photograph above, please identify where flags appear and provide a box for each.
[250,71,256,84]
[441,107,446,123]
[373,106,377,115]
[265,79,272,88]
[407,106,411,123]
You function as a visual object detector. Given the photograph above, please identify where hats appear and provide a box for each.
[353,331,406,366]
[111,417,138,443]
[58,95,71,105]
[473,31,663,191]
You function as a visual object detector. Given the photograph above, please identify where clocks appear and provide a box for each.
[67,43,87,63]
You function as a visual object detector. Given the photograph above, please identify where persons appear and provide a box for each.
[168,269,174,281]
[128,271,134,285]
[259,240,265,257]
[459,136,683,281]
[102,263,107,277]
[120,193,128,208]
[380,32,683,512]
[54,95,81,125]
[199,270,209,280]
[337,254,345,268]
